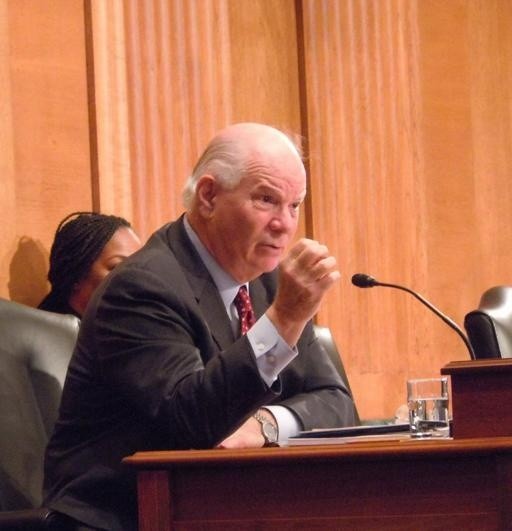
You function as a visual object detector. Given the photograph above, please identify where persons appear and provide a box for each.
[36,210,148,324]
[34,118,360,531]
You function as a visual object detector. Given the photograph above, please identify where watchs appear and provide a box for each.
[252,414,279,445]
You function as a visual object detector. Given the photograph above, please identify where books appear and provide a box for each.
[288,420,448,447]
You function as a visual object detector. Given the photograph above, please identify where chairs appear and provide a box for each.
[0,298,80,531]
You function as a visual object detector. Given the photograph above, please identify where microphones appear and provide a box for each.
[351,273,477,360]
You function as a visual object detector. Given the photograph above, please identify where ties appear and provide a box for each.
[233,284,257,336]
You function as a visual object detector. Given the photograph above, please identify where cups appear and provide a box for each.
[407,378,450,440]
